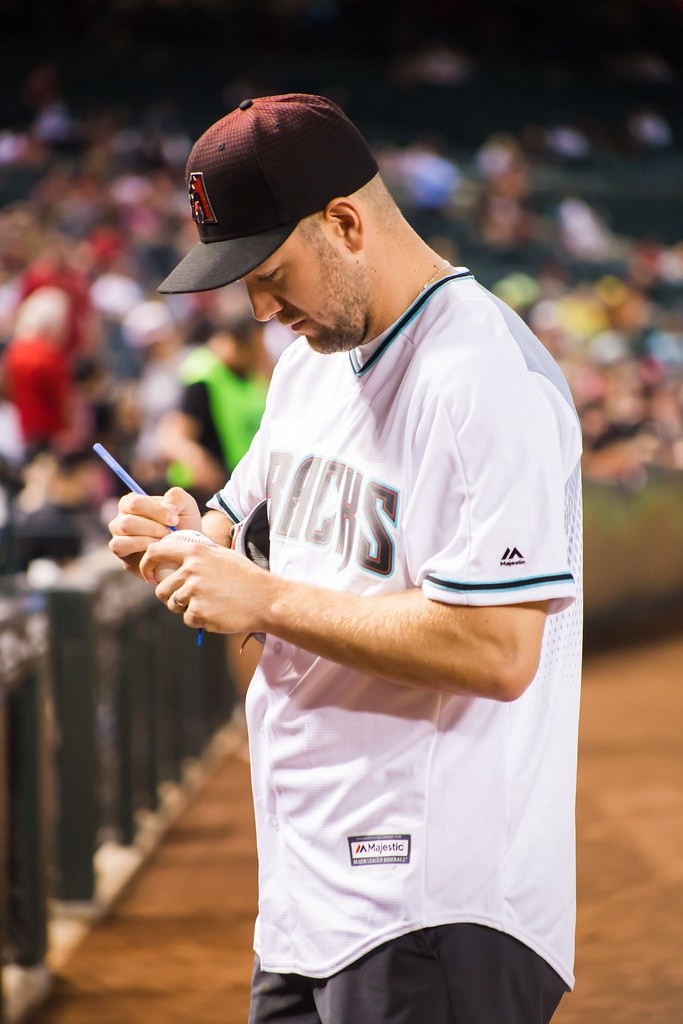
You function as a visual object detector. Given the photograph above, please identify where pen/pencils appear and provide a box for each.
[92,441,178,532]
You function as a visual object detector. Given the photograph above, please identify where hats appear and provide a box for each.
[156,93,380,293]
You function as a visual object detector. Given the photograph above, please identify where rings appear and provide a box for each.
[173,592,187,608]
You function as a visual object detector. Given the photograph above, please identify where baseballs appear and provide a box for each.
[155,529,208,581]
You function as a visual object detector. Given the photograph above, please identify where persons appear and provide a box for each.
[107,93,582,1024]
[0,64,682,908]
